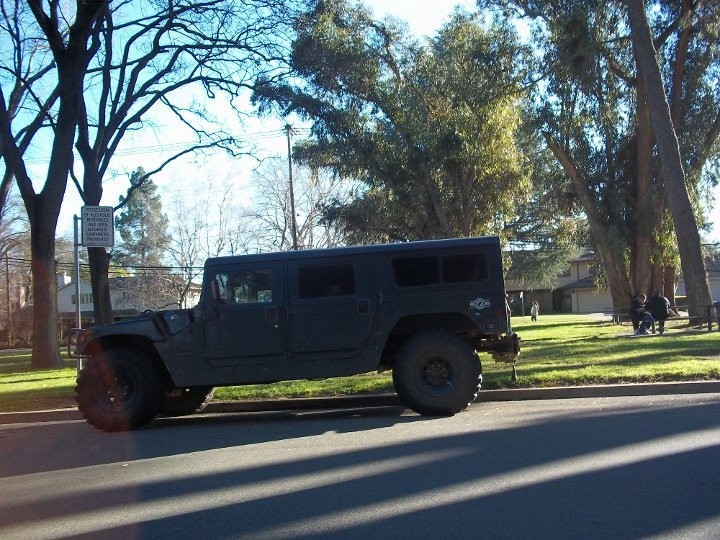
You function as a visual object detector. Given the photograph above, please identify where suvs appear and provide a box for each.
[69,235,520,434]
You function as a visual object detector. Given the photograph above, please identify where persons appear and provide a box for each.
[629,293,652,335]
[531,301,539,322]
[262,293,272,303]
[645,291,671,334]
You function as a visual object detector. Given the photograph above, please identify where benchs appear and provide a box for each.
[604,306,631,326]
[652,315,703,333]
